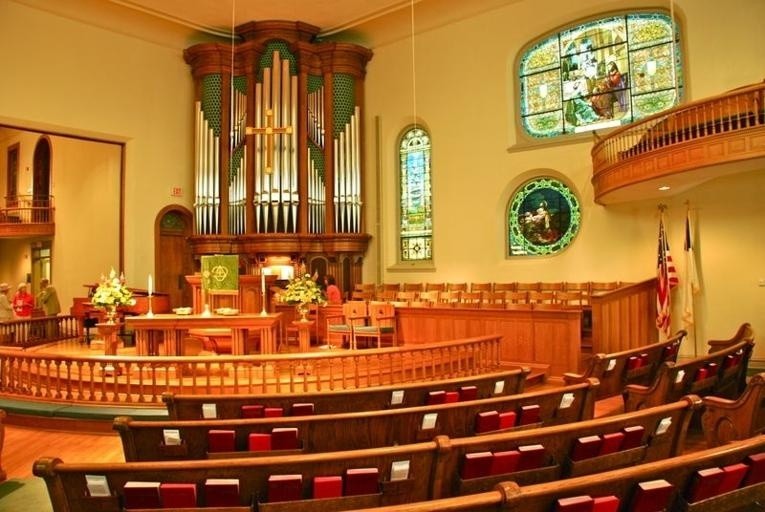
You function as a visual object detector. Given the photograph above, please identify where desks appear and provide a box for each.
[125,313,283,355]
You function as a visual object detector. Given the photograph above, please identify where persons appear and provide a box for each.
[323,275,342,304]
[522,202,549,232]
[12,283,36,344]
[36,278,61,340]
[607,60,628,113]
[0,282,17,343]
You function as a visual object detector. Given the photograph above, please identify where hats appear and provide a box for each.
[0,283,12,291]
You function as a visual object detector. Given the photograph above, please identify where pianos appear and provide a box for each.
[71,284,170,345]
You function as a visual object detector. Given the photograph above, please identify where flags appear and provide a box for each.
[655,211,699,345]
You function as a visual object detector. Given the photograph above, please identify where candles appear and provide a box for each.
[148,273,153,295]
[261,271,265,295]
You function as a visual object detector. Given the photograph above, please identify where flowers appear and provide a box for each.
[90,266,137,312]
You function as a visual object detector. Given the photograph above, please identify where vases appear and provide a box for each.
[104,305,117,324]
